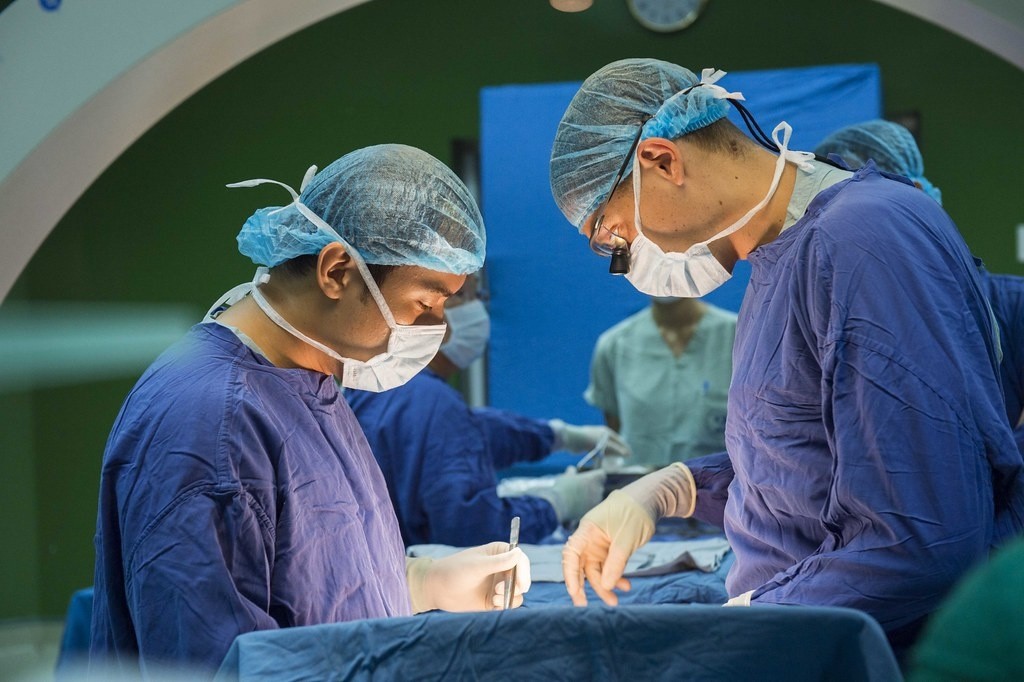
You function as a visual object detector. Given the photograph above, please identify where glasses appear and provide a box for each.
[587,115,649,276]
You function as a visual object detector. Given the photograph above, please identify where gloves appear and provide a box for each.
[404,541,531,616]
[547,417,635,458]
[721,587,756,607]
[561,462,697,609]
[533,464,611,525]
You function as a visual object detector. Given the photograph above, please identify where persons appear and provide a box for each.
[551,58,1023,653]
[88,143,531,682]
[343,282,630,548]
[583,294,736,469]
[812,118,1024,442]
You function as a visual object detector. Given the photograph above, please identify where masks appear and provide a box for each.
[440,299,490,371]
[621,163,732,299]
[335,243,447,393]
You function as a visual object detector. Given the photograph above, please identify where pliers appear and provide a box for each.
[577,433,610,472]
